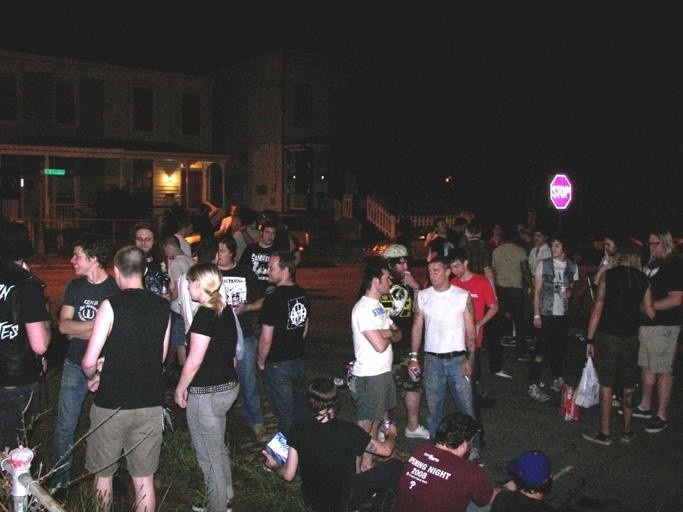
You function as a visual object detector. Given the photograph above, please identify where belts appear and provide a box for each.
[427,351,465,359]
[189,380,238,394]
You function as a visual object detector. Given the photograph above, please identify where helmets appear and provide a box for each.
[383,244,409,260]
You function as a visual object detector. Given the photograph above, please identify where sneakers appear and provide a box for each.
[540,377,565,392]
[582,430,613,446]
[255,426,268,442]
[405,424,430,440]
[468,447,485,467]
[620,431,637,442]
[492,368,513,381]
[192,499,233,512]
[528,384,552,403]
[645,416,669,433]
[377,420,391,443]
[495,336,544,363]
[612,395,653,419]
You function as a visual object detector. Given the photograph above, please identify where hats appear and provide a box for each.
[507,450,551,486]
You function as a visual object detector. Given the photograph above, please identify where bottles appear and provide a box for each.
[159,277,170,294]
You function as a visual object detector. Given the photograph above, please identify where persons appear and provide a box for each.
[2,199,312,511]
[350,217,683,472]
[272,378,559,511]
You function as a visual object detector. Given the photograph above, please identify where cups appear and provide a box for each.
[558,285,566,295]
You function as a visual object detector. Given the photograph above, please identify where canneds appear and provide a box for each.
[383,421,391,440]
[410,368,420,383]
